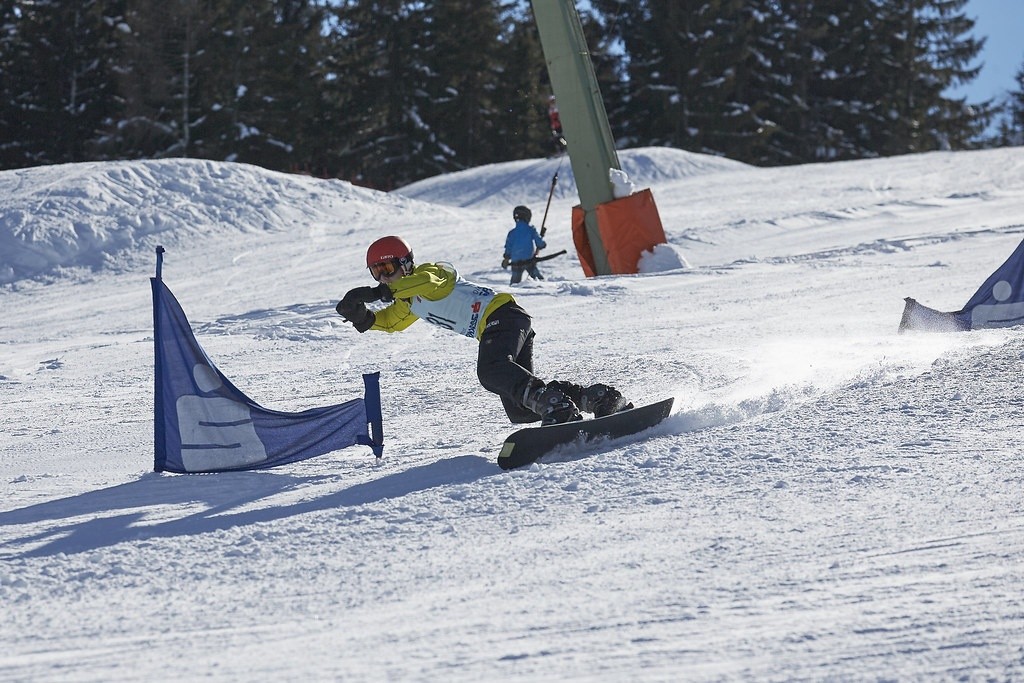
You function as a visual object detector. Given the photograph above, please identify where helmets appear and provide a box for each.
[366,236,412,267]
[513,206,532,223]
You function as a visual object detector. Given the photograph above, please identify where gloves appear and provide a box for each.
[336,300,376,335]
[502,259,508,268]
[343,284,393,304]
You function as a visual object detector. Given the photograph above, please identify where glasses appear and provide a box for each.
[369,251,412,280]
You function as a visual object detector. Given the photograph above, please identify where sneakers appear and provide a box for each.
[528,387,583,428]
[581,384,627,418]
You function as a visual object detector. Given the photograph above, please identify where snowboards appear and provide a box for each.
[497,396,676,471]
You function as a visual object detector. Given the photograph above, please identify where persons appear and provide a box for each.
[502,206,546,286]
[336,236,634,428]
